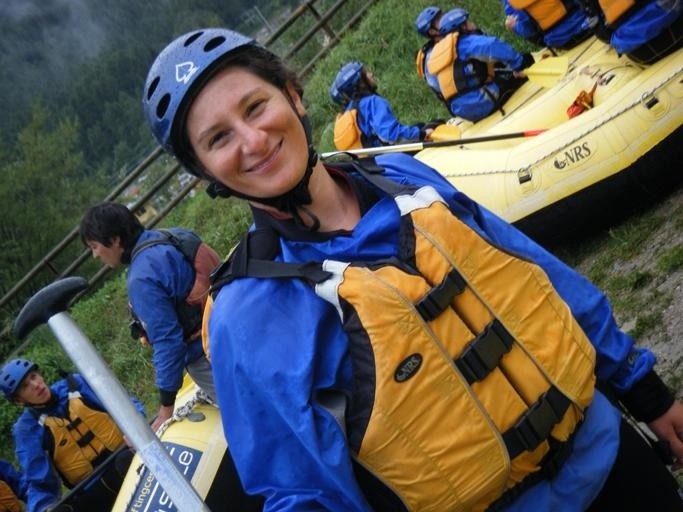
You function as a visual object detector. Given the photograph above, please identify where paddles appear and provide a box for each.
[491,55,569,87]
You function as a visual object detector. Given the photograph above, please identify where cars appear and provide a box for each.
[176,173,193,188]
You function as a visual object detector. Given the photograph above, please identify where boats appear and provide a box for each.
[41,375,265,512]
[412,31,682,244]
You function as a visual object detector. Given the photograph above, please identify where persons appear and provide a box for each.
[0,458,30,510]
[329,0,682,158]
[141,28,680,511]
[0,357,148,511]
[79,203,225,436]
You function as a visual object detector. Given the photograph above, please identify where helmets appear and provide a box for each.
[0,358,38,398]
[142,27,281,182]
[331,6,469,106]
